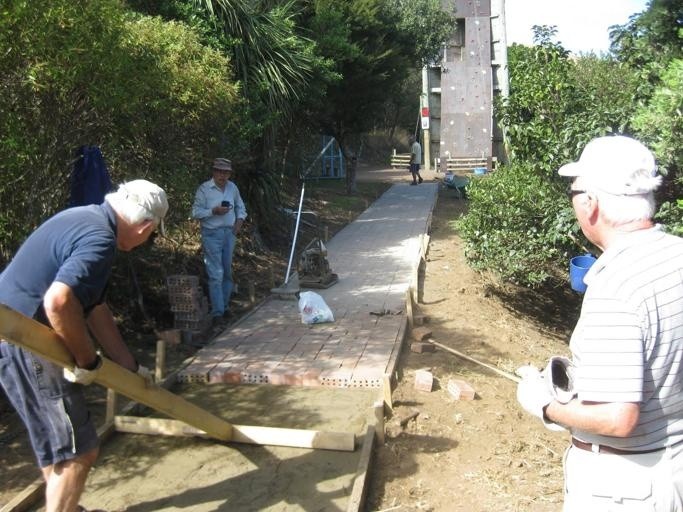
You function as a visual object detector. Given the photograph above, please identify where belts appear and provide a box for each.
[572,437,682,455]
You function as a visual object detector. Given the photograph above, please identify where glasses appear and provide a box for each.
[566,184,591,202]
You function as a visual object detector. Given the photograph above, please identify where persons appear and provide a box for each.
[407,134,422,186]
[190,157,246,327]
[514,136,682,510]
[0,178,169,511]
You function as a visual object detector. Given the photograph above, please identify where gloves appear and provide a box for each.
[539,357,577,404]
[64,355,102,386]
[135,365,153,387]
[516,367,565,430]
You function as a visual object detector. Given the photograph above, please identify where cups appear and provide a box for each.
[221,200,233,213]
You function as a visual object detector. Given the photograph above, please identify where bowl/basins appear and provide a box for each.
[473,168,487,174]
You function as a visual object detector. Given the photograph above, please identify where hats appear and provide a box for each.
[214,158,231,171]
[120,180,168,236]
[558,136,655,196]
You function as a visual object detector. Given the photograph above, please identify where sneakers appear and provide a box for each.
[411,182,417,185]
[224,310,231,317]
[419,177,423,183]
[213,316,223,324]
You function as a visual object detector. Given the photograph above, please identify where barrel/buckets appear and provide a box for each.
[474,167,487,174]
[444,172,455,188]
